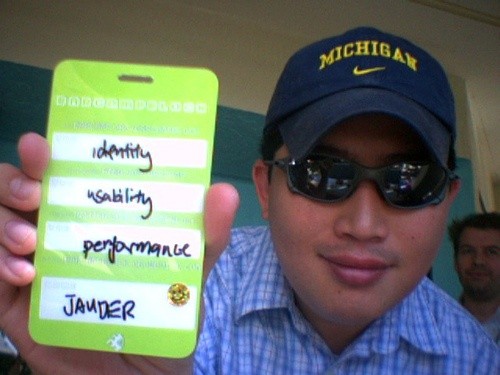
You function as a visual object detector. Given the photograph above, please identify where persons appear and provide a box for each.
[1,27,499,374]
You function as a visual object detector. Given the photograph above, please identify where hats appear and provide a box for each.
[261,26,457,177]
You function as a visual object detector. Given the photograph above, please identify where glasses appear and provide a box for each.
[264,151,454,209]
